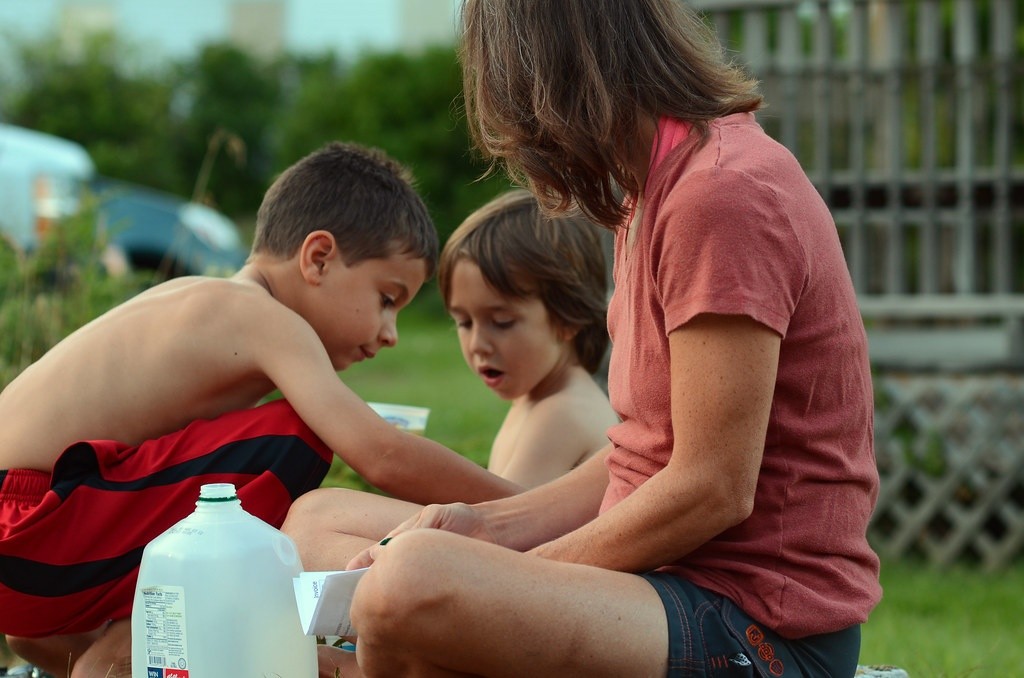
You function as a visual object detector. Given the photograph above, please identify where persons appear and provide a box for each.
[282,1,879,678]
[0,142,525,678]
[439,188,616,487]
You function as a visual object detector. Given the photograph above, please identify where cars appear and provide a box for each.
[68,166,247,290]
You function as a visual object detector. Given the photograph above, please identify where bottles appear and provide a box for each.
[130,484,319,678]
